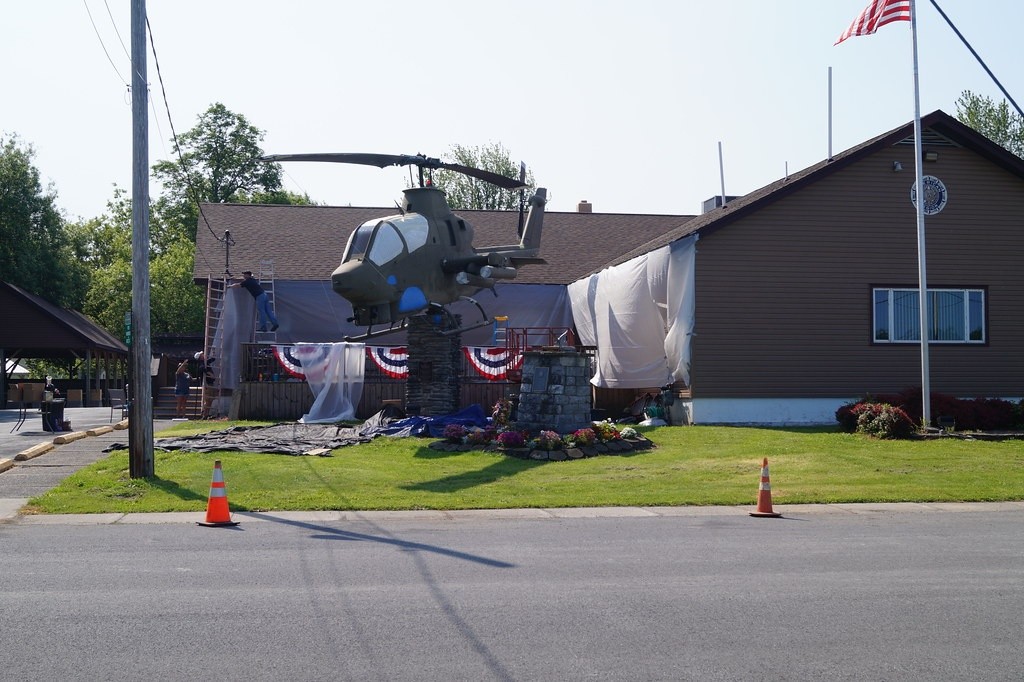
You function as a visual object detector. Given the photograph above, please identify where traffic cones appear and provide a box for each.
[748,457,781,516]
[196,461,241,526]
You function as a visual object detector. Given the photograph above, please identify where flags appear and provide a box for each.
[832,0,912,46]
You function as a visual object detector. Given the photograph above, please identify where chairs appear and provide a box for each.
[107,389,129,422]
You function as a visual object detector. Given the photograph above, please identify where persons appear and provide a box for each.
[174,359,192,418]
[194,351,216,386]
[227,271,279,332]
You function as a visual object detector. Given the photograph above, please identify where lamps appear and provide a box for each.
[894,162,903,172]
[925,153,938,162]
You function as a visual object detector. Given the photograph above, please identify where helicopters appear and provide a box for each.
[257,152,550,343]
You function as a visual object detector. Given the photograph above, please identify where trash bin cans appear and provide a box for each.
[41,398,65,431]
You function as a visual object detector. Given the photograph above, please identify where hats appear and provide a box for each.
[194,351,203,360]
[242,271,251,276]
[178,363,188,367]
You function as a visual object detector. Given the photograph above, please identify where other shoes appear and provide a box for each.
[271,324,279,332]
[256,328,267,332]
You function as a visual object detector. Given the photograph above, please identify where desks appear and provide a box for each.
[8,399,56,435]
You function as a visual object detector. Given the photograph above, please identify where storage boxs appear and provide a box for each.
[7,382,46,403]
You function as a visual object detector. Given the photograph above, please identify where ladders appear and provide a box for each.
[200,272,228,419]
[253,264,279,344]
[493,316,511,346]
